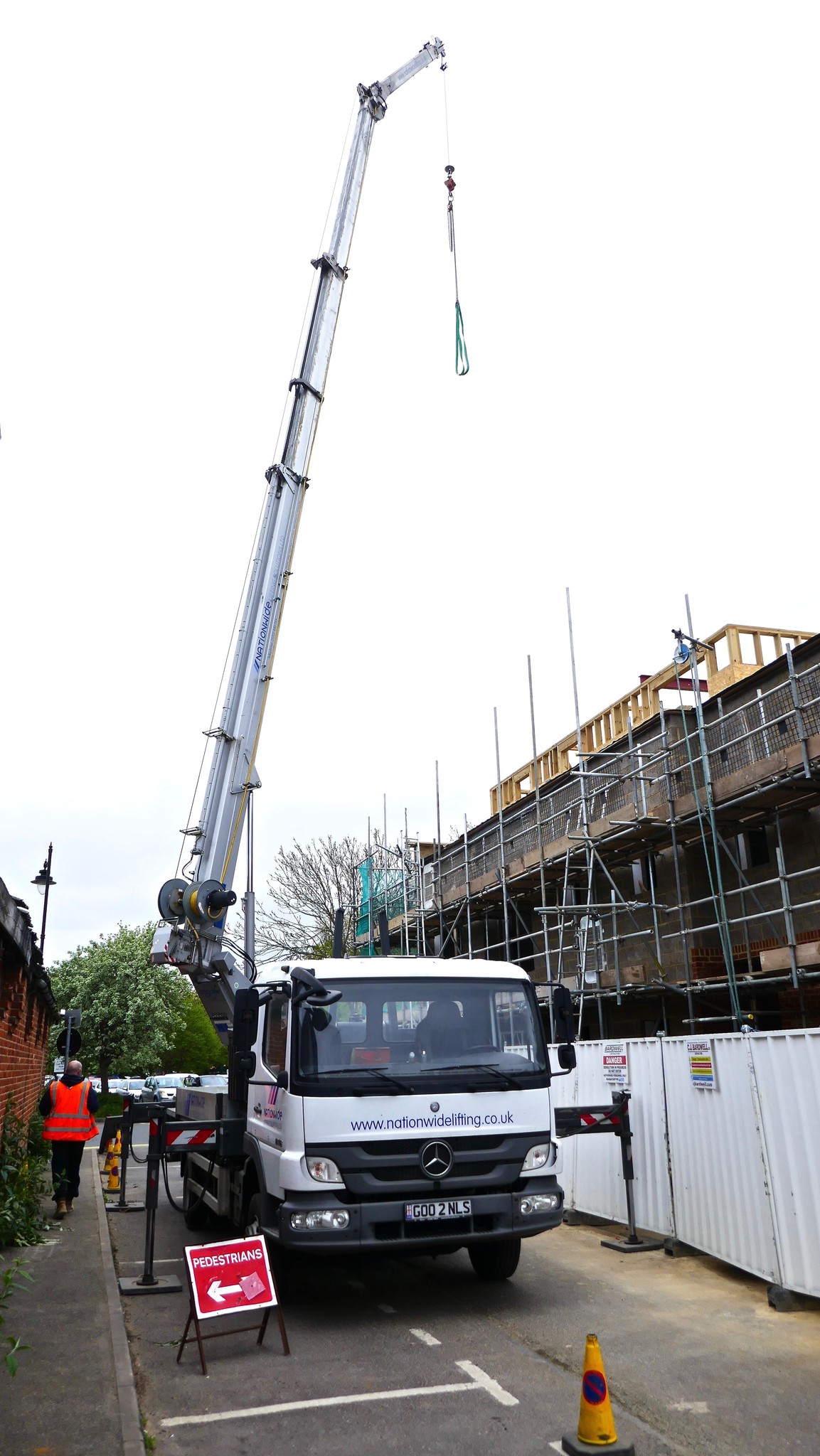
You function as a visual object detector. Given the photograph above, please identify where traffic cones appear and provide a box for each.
[561,1333,635,1456]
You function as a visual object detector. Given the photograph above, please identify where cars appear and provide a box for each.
[183,1075,228,1088]
[139,1075,187,1106]
[115,1079,146,1103]
[44,1073,64,1088]
[163,1073,199,1085]
[89,1078,123,1093]
[217,1074,228,1084]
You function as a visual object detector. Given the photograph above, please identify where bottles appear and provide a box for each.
[421,1051,427,1063]
[408,1052,415,1063]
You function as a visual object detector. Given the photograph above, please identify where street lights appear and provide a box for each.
[29,840,57,966]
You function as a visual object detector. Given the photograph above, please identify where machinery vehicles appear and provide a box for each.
[97,35,666,1305]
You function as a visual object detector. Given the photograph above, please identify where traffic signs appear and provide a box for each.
[183,1234,278,1320]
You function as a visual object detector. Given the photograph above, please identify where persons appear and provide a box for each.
[182,1075,196,1086]
[38,1060,99,1218]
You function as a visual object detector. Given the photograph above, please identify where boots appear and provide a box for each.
[54,1198,67,1220]
[67,1201,74,1213]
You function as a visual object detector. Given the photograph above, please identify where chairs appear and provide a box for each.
[414,1003,475,1057]
[312,1023,343,1065]
